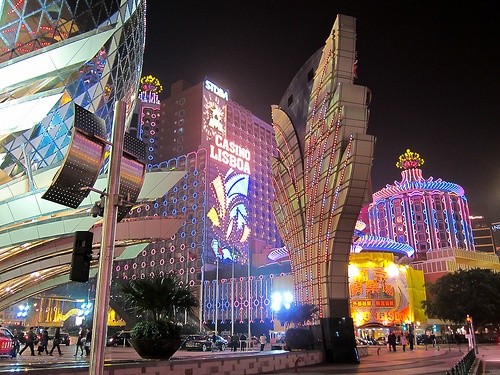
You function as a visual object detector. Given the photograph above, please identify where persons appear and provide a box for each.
[112,330,121,347]
[18,327,36,356]
[178,331,246,352]
[0,321,24,345]
[48,328,63,356]
[74,325,92,356]
[259,333,267,352]
[37,327,51,355]
[279,334,286,351]
[359,329,462,352]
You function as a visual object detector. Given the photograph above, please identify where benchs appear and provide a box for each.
[358,346,387,355]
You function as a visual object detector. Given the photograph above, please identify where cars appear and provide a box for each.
[355,336,389,346]
[21,331,39,345]
[58,333,71,346]
[179,334,258,352]
[0,328,20,357]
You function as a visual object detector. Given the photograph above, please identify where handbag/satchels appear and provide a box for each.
[37,346,44,352]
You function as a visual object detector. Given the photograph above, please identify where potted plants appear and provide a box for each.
[128,321,185,360]
